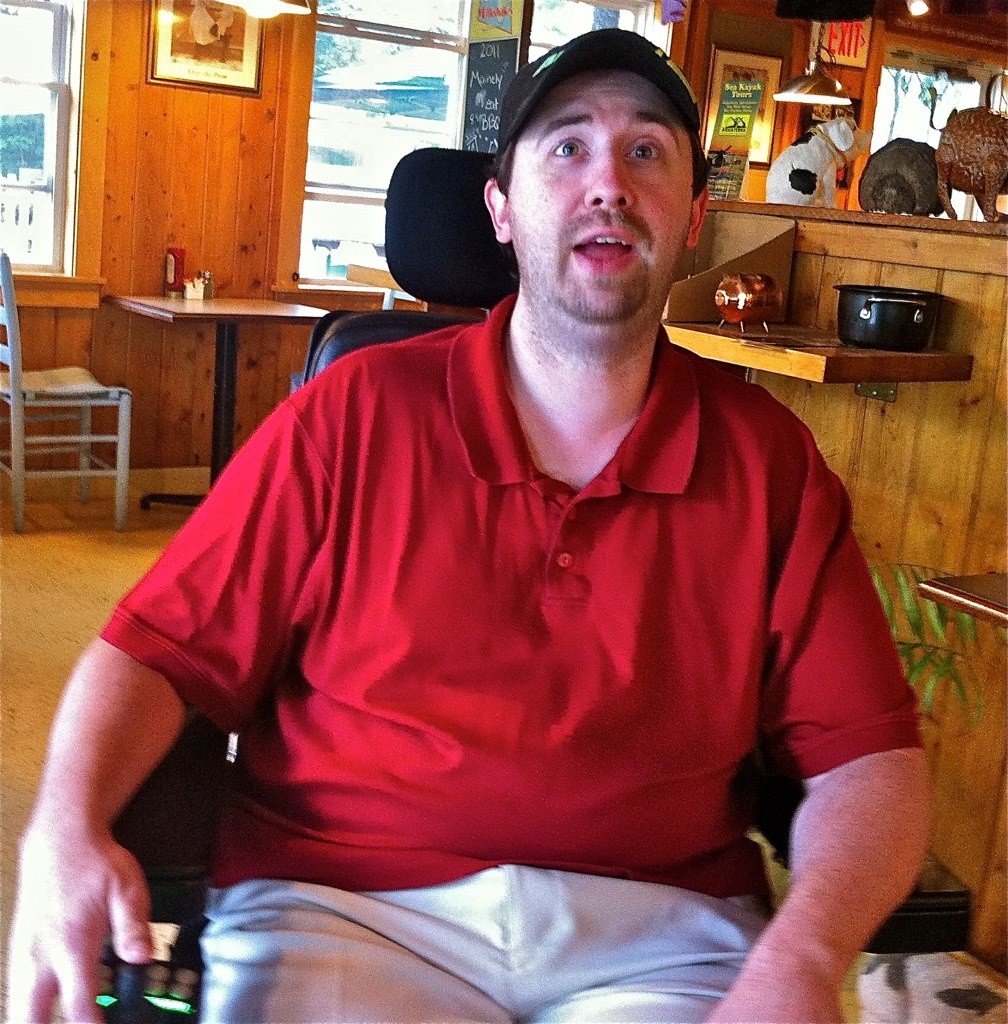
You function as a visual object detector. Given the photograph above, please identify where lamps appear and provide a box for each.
[772,20,854,109]
[212,0,314,23]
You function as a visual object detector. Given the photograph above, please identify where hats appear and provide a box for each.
[495,27,701,158]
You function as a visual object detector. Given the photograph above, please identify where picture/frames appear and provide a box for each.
[143,0,266,101]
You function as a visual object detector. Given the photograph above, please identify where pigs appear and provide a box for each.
[715,271,781,334]
[764,114,873,209]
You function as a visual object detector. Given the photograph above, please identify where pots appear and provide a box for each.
[832,285,946,353]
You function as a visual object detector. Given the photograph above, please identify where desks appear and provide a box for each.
[103,292,334,511]
[666,314,973,387]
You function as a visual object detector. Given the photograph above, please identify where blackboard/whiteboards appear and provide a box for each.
[455,36,521,152]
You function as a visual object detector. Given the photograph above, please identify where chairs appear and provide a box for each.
[91,145,976,1024]
[0,245,134,534]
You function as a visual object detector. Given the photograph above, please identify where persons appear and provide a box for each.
[8,25,938,1024]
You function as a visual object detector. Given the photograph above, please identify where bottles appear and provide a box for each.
[164,247,186,299]
[200,271,214,299]
